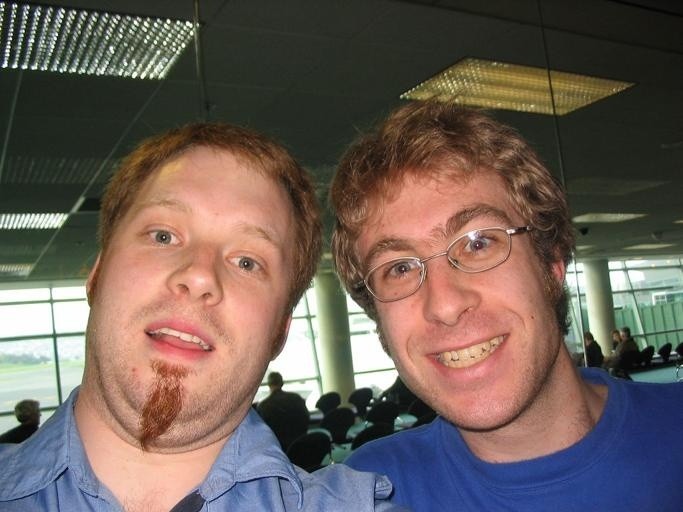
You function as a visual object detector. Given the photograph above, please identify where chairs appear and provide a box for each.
[639,342,683,365]
[282,386,436,475]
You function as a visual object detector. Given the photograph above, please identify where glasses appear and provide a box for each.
[353,226,535,303]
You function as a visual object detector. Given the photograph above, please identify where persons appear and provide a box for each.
[0,399,42,443]
[256,372,309,452]
[327,102,683,512]
[582,327,641,368]
[0,122,394,511]
